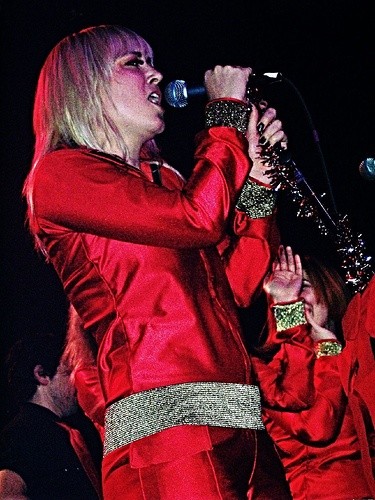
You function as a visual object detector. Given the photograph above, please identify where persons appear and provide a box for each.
[250,245,375,500]
[22,26,287,500]
[0,334,104,500]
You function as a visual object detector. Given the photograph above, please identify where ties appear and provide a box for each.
[57,422,102,499]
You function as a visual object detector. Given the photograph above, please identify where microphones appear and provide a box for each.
[165,71,283,107]
[359,158,375,180]
